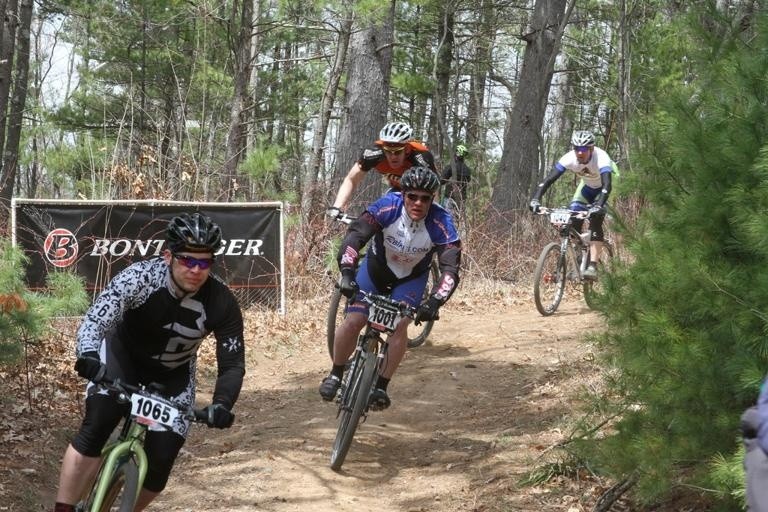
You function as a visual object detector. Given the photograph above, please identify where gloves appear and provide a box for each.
[78,355,100,379]
[530,199,541,212]
[196,402,236,429]
[414,298,438,325]
[340,277,359,297]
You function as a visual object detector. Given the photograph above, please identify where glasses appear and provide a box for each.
[575,146,587,151]
[407,194,432,201]
[172,253,215,269]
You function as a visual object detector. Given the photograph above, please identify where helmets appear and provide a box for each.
[164,213,222,253]
[457,145,467,157]
[570,130,595,146]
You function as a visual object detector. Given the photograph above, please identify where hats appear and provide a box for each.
[379,120,440,193]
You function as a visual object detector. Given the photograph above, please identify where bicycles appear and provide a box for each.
[72,354,233,512]
[327,206,439,372]
[325,269,439,471]
[527,201,616,315]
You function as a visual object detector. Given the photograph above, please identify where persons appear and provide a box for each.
[738,374,768,512]
[529,130,621,281]
[330,121,437,224]
[319,165,464,412]
[52,209,247,510]
[440,144,472,214]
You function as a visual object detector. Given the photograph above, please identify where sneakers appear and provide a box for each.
[371,390,391,408]
[583,269,598,279]
[319,377,339,400]
